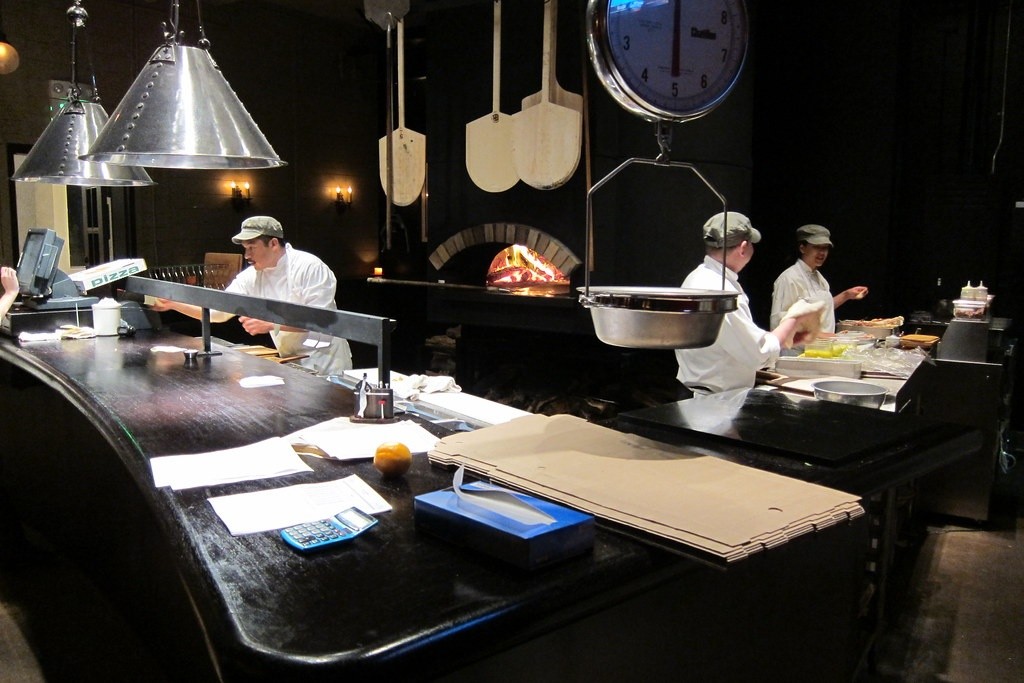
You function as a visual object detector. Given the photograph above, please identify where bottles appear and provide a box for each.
[960,280,974,301]
[885,334,900,349]
[975,281,988,302]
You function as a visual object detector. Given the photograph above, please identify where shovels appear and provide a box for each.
[466,0,520,192]
[379,18,426,207]
[509,0,583,190]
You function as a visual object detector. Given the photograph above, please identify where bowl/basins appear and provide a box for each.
[812,381,890,412]
[572,286,742,350]
[804,330,868,358]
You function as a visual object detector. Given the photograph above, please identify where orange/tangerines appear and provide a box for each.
[373,441,412,478]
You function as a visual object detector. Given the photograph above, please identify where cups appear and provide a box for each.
[91,304,122,336]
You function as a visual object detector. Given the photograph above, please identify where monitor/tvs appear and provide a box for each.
[16,228,65,296]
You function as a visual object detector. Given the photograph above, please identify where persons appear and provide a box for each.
[771,225,869,349]
[153,214,353,376]
[674,211,825,400]
[0,267,20,325]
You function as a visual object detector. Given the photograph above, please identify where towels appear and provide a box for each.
[390,374,463,403]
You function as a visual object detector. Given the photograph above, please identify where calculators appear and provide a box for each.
[281,507,379,553]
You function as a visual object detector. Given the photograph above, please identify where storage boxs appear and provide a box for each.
[68,258,147,292]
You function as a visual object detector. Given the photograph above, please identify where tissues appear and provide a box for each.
[411,460,598,574]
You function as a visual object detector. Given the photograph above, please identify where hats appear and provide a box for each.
[797,224,833,247]
[702,212,761,247]
[232,216,283,244]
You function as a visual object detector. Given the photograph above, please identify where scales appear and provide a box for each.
[577,1,743,350]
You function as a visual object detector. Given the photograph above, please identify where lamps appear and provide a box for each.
[330,185,354,216]
[229,179,253,213]
[0,18,20,75]
